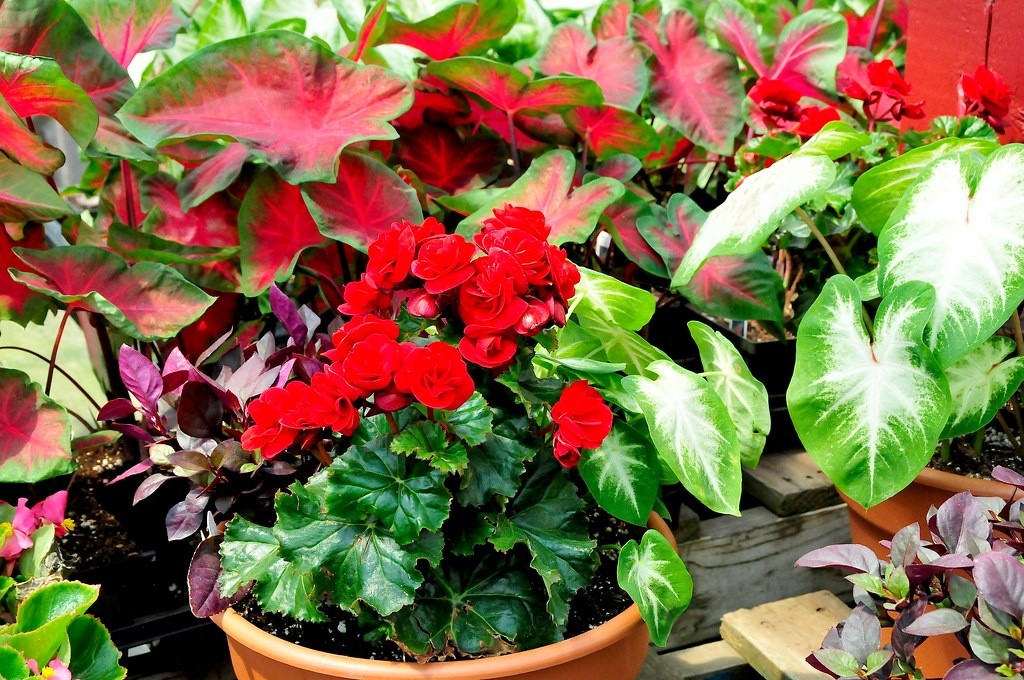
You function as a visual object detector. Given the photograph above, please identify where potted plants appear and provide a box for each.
[0,0,418,680]
[526,0,906,455]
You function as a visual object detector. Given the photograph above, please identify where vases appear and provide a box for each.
[836,468,1024,680]
[210,510,678,680]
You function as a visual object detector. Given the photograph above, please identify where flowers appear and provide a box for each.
[218,200,772,666]
[666,52,1024,511]
